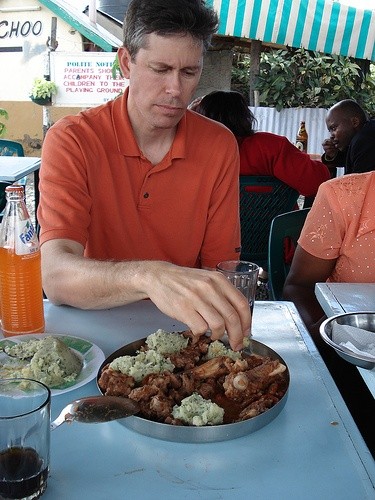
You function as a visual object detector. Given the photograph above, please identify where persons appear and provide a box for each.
[37,0,252,351]
[278,169,375,345]
[184,90,334,265]
[321,100,375,177]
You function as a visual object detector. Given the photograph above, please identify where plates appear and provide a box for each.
[1,334,103,400]
[96,329,289,443]
[319,310,375,368]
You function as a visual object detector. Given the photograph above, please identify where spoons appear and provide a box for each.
[52,394,137,433]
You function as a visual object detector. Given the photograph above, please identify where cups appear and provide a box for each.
[216,259,258,322]
[0,380,53,500]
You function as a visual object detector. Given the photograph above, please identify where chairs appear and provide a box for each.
[268,207,312,301]
[238,176,299,300]
[0,140,24,158]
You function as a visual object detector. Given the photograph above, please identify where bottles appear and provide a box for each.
[295,121,307,154]
[0,184,46,338]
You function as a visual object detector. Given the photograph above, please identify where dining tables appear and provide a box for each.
[314,284,375,397]
[0,296,375,500]
[0,154,41,230]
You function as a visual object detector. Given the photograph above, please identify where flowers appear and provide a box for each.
[32,78,56,100]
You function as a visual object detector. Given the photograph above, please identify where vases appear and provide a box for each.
[30,95,51,105]
